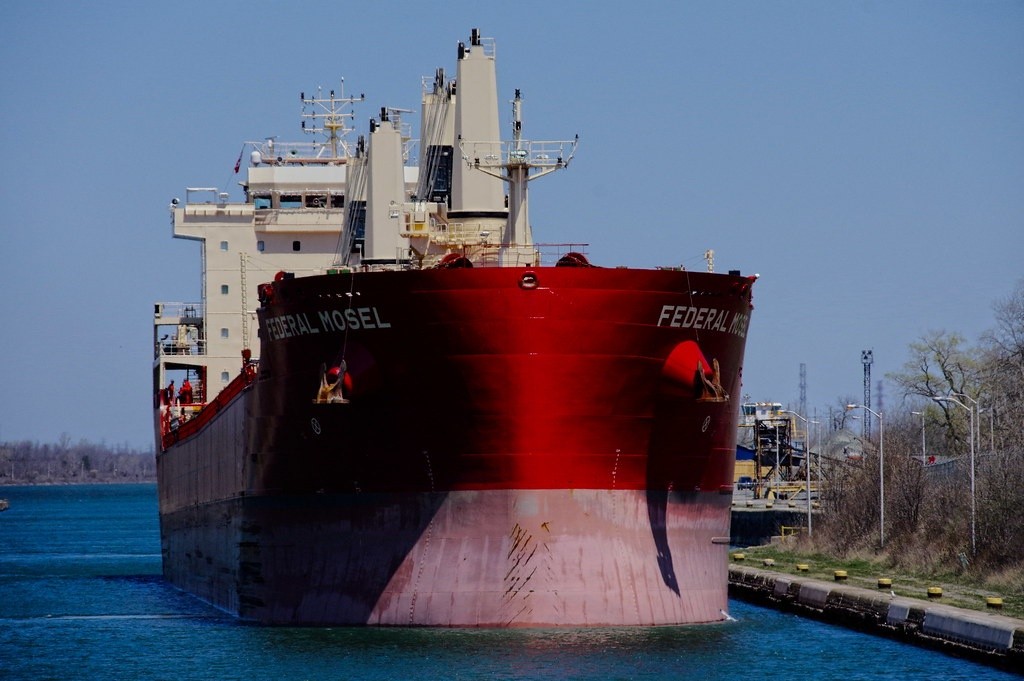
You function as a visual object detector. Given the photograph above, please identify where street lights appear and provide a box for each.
[932,396,976,557]
[951,393,980,452]
[852,414,865,468]
[912,411,926,466]
[847,404,883,548]
[810,421,821,499]
[780,410,812,536]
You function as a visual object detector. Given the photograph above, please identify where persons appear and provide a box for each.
[167,378,193,404]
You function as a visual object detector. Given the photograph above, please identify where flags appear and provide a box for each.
[234,159,240,173]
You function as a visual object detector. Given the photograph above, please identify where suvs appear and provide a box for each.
[738,476,753,490]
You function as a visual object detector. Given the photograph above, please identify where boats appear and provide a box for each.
[736,402,806,466]
[153,27,760,628]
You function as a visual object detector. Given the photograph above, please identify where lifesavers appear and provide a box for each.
[440,254,473,268]
[274,271,286,280]
[556,252,589,268]
